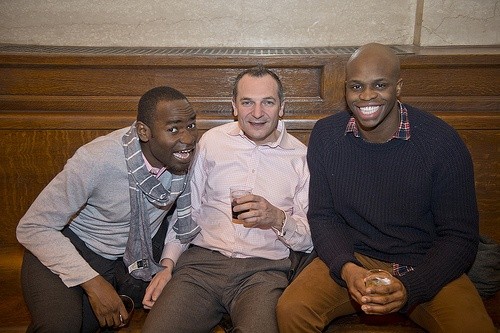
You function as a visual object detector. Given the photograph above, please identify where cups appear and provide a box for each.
[365,269,394,290]
[230,185,253,224]
[110,295,135,329]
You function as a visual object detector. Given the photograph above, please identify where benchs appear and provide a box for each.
[0,112,500,333]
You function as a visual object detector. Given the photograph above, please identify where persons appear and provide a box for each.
[142,68,313,333]
[275,43,497,332]
[15,86,202,333]
